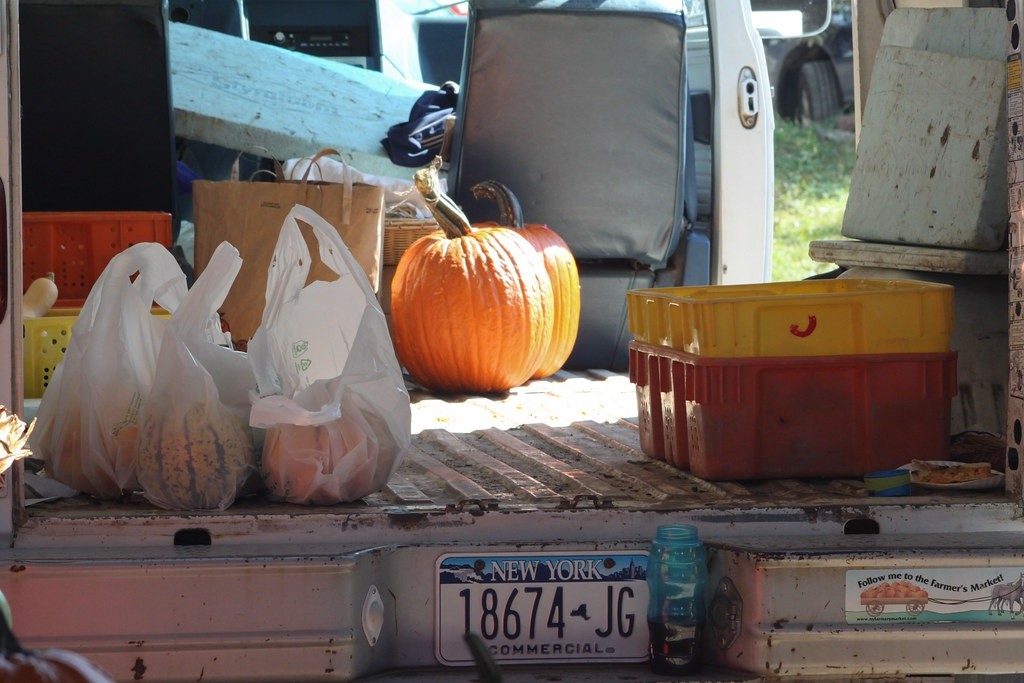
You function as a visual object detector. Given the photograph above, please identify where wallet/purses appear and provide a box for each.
[22,209,172,306]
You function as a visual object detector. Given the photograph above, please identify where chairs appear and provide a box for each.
[450,0,694,367]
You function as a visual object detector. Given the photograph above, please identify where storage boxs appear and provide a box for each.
[22,210,195,399]
[627,279,959,477]
[0,540,1024,682]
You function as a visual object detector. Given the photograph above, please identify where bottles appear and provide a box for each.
[644,524,710,677]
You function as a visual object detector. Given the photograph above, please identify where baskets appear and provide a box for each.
[382,217,442,267]
[20,306,170,400]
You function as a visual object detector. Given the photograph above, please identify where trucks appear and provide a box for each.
[0,1,1024,683]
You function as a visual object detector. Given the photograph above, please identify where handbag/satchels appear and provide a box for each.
[247,204,412,505]
[193,145,384,352]
[131,240,265,511]
[26,242,187,501]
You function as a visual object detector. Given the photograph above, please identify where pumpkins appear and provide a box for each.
[44,160,581,512]
